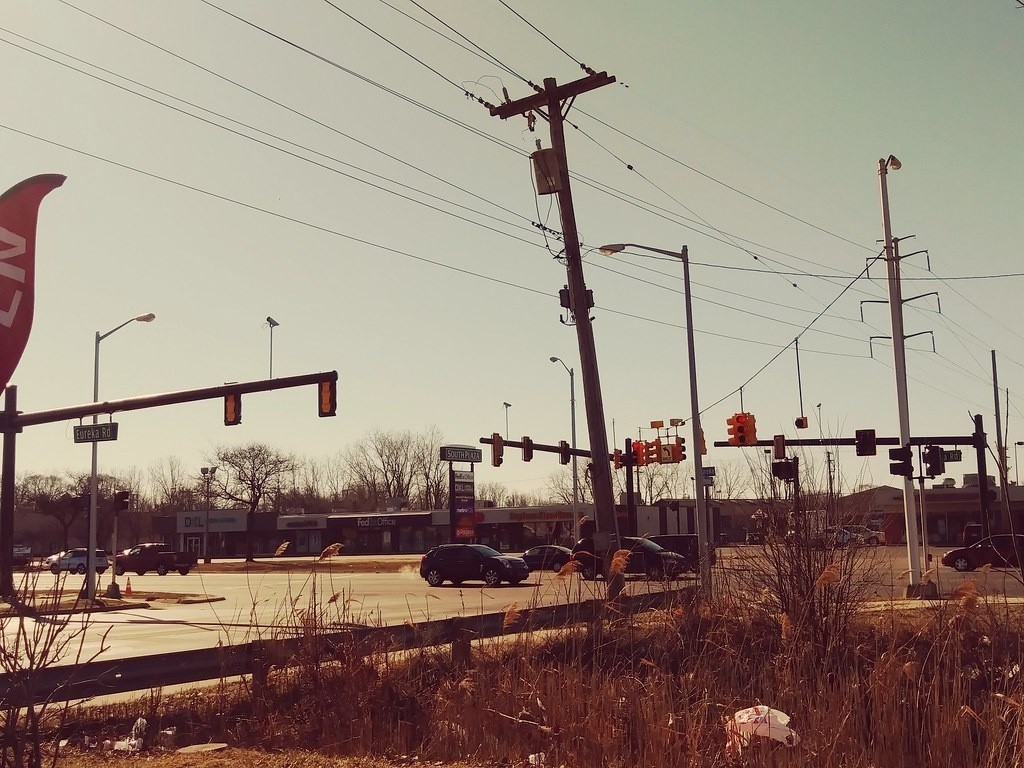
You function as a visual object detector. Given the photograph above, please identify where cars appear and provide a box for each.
[12,543,32,566]
[784,524,886,552]
[518,544,572,573]
[941,533,1024,572]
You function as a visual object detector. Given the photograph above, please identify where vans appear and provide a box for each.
[962,522,982,547]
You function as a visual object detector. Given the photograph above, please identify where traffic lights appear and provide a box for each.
[114,491,129,511]
[651,439,661,462]
[726,416,738,445]
[923,445,947,475]
[645,442,653,466]
[795,417,808,428]
[734,414,750,445]
[633,442,640,466]
[614,449,622,469]
[676,437,686,462]
[889,442,914,477]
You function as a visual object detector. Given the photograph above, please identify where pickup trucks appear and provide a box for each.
[115,542,199,576]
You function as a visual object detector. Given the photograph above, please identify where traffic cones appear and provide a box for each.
[123,576,135,597]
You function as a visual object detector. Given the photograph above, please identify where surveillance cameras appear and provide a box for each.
[503,402,511,407]
[266,317,280,326]
[817,403,822,407]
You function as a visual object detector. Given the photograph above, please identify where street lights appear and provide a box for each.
[201,467,219,564]
[550,356,581,546]
[597,243,712,602]
[1014,442,1024,487]
[86,312,156,600]
[877,154,922,598]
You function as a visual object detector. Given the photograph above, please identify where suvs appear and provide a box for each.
[420,544,530,589]
[645,533,717,574]
[570,533,691,583]
[50,547,109,575]
